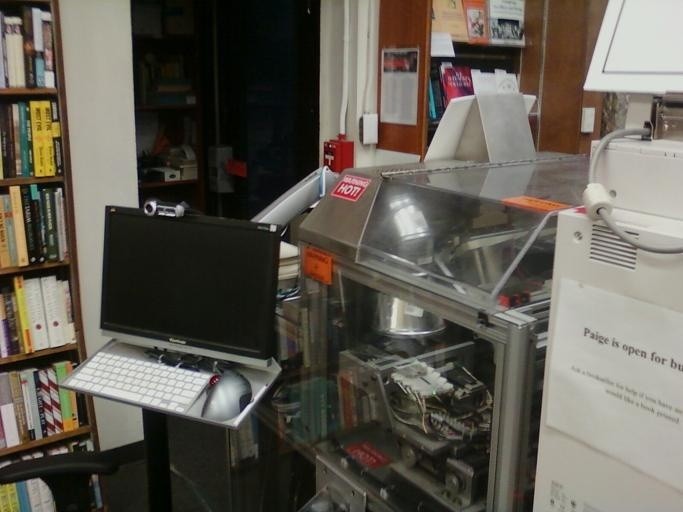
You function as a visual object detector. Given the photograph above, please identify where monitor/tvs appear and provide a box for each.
[583,0,683,94]
[99,204,282,369]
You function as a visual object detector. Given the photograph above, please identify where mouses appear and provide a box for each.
[200,368,252,422]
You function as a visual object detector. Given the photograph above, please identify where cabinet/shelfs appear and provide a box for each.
[0,1,101,459]
[375,0,538,160]
[166,147,587,512]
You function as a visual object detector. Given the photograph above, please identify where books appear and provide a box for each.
[428,0,525,121]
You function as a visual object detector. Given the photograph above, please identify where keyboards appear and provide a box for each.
[67,351,217,414]
